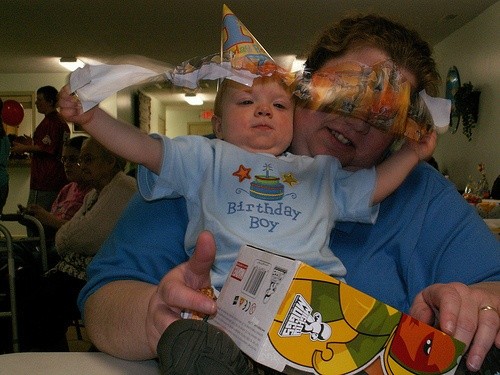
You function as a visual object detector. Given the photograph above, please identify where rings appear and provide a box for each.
[480,306,497,313]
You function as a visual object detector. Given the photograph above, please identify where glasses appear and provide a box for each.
[59,155,75,165]
[77,153,105,167]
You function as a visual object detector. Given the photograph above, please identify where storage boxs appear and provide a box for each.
[205,242,467,375]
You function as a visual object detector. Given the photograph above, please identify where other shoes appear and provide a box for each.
[156,319,265,375]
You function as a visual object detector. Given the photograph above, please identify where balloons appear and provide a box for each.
[1,99,24,126]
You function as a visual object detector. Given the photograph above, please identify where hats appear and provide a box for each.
[220,4,304,91]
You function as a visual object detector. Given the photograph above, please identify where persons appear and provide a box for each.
[76,14,500,375]
[0,135,94,312]
[0,137,138,356]
[57,58,500,375]
[9,86,71,238]
